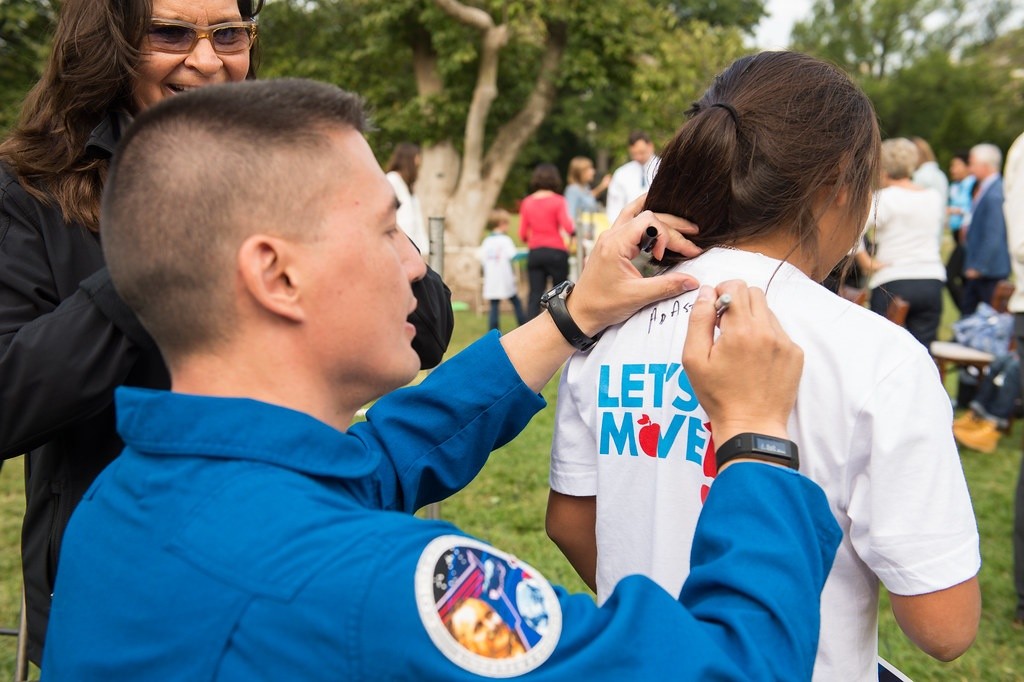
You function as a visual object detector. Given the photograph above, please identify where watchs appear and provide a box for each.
[541,281,597,353]
[714,433,799,473]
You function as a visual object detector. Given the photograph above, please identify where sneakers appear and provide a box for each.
[954,413,1003,453]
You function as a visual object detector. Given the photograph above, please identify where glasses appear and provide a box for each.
[143,17,257,55]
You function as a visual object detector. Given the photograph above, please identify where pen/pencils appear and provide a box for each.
[714,292,732,317]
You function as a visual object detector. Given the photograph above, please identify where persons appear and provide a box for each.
[40,79,843,682]
[0,0,453,667]
[831,131,1024,626]
[545,50,980,682]
[478,207,526,333]
[519,164,577,321]
[562,155,611,227]
[606,131,663,225]
[383,142,429,267]
